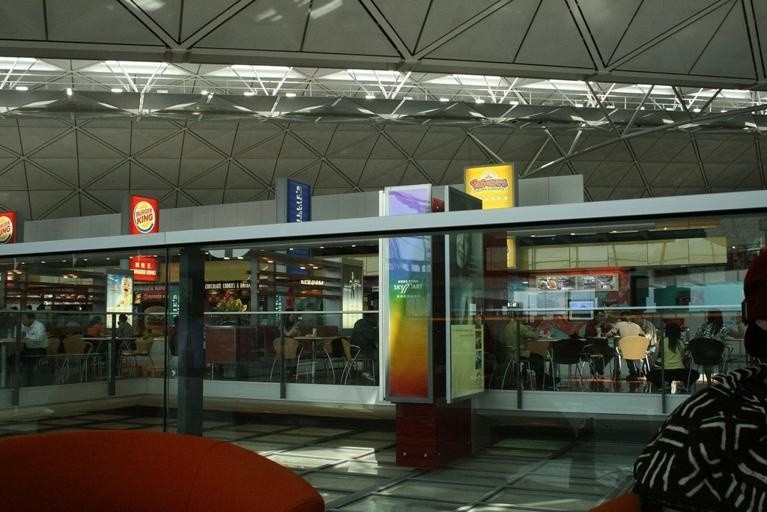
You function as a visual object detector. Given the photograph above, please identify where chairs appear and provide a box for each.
[167,336,179,378]
[270,323,379,386]
[42,319,157,385]
[483,320,734,391]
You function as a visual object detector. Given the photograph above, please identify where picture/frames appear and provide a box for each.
[596,274,620,293]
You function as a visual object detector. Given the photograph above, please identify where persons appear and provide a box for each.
[86,315,104,337]
[503,310,745,390]
[632,242,767,511]
[115,314,133,348]
[168,314,179,375]
[283,307,302,338]
[20,312,48,364]
[350,314,378,380]
